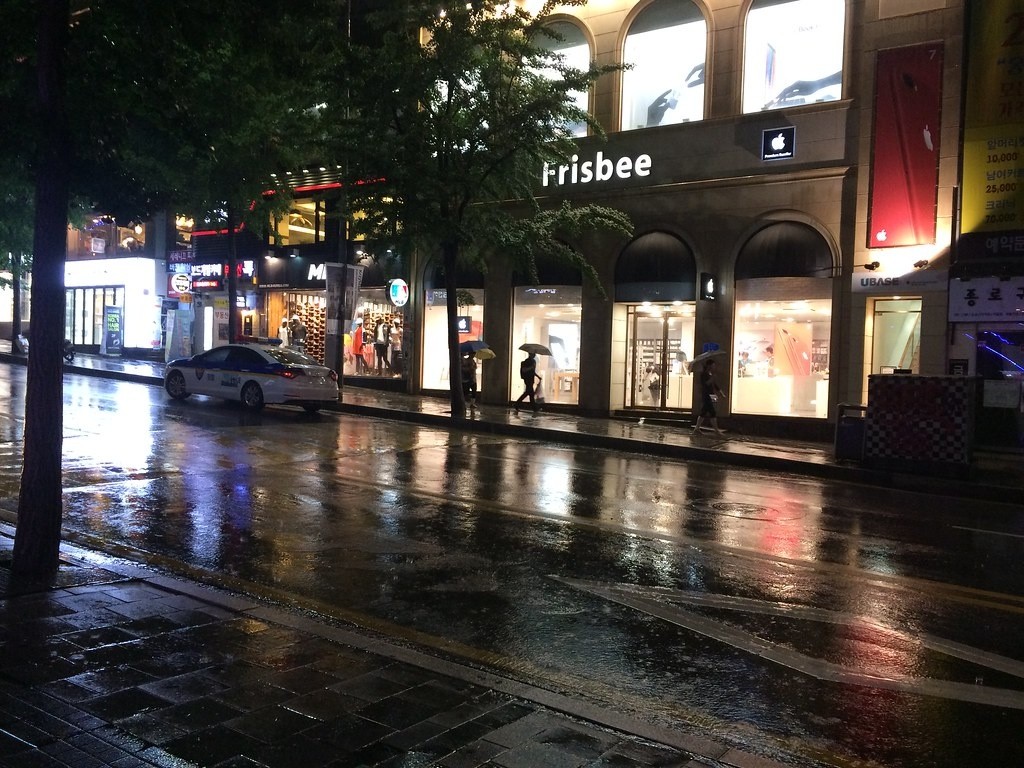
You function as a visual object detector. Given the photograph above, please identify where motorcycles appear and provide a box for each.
[63,338,75,362]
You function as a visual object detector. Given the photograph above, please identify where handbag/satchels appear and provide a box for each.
[535,380,543,395]
[708,394,719,403]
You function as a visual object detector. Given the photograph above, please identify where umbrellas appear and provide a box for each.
[460,340,490,355]
[519,343,552,364]
[687,350,726,374]
[475,349,496,359]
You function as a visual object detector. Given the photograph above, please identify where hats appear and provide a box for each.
[291,315,299,319]
[394,318,400,324]
[282,318,288,323]
[374,315,382,320]
[355,317,364,324]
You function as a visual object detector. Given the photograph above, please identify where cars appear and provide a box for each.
[163,335,339,414]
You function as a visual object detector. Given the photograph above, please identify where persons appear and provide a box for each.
[512,353,542,412]
[373,315,391,376]
[388,318,403,378]
[738,345,774,377]
[645,364,660,406]
[462,352,478,408]
[694,361,725,435]
[276,318,291,348]
[352,318,372,376]
[290,315,307,355]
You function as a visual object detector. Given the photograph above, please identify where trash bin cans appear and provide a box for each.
[834,402,868,467]
